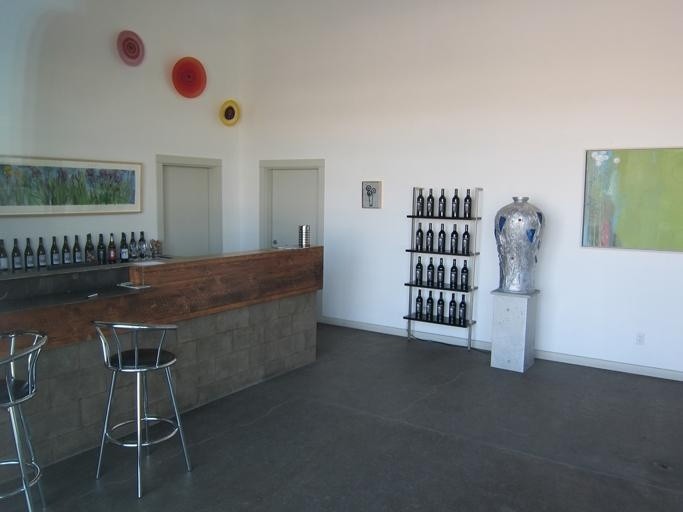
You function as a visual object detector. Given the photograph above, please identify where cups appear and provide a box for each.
[298,224,310,247]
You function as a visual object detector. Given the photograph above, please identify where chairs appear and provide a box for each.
[0,329,51,512]
[91,316,193,498]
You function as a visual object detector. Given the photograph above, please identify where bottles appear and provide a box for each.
[50,236,60,265]
[438,189,446,218]
[24,237,34,268]
[108,233,116,264]
[448,293,456,325]
[460,260,469,291]
[450,224,458,254]
[449,259,458,290]
[437,257,444,288]
[137,230,147,258]
[415,222,423,251]
[461,224,470,256]
[451,189,459,218]
[437,292,444,323]
[129,232,137,258]
[97,233,106,264]
[426,189,434,216]
[73,234,82,264]
[458,294,467,327]
[464,189,471,218]
[84,233,94,264]
[37,236,46,268]
[0,239,9,271]
[12,239,22,269]
[426,290,434,321]
[62,236,71,264]
[416,189,424,216]
[120,232,129,263]
[427,257,434,287]
[437,224,446,253]
[415,255,423,286]
[415,290,423,319]
[425,223,434,252]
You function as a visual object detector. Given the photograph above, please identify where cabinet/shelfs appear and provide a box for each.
[404,185,484,351]
[0,257,139,313]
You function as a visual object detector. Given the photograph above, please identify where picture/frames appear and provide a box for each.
[361,181,383,208]
[0,157,145,217]
